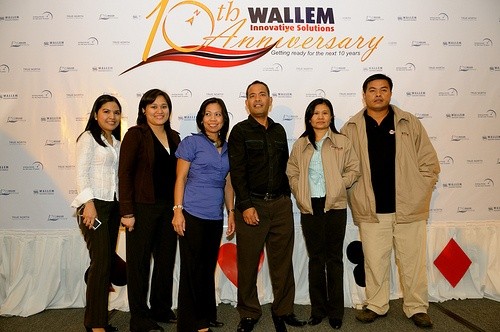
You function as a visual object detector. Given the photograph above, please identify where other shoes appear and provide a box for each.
[130,319,164,332]
[86,325,118,332]
[150,308,178,323]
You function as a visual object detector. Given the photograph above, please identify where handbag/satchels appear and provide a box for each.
[84,251,127,286]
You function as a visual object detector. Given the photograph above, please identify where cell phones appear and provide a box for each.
[92,217,102,231]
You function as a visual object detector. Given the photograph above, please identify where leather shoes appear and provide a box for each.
[329,319,342,329]
[193,327,213,332]
[208,320,224,327]
[307,314,327,325]
[279,315,307,327]
[237,317,256,332]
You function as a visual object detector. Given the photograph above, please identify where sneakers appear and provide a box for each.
[411,313,434,329]
[355,308,388,323]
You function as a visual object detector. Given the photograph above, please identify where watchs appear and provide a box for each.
[227,209,235,213]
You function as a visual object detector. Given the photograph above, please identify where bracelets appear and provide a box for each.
[122,215,134,218]
[172,204,183,210]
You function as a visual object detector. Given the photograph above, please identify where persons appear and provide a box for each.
[118,88,181,332]
[285,97,361,330]
[228,80,308,332]
[340,73,440,328]
[70,95,121,332]
[171,98,234,332]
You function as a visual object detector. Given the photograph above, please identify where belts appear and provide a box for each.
[251,192,283,202]
